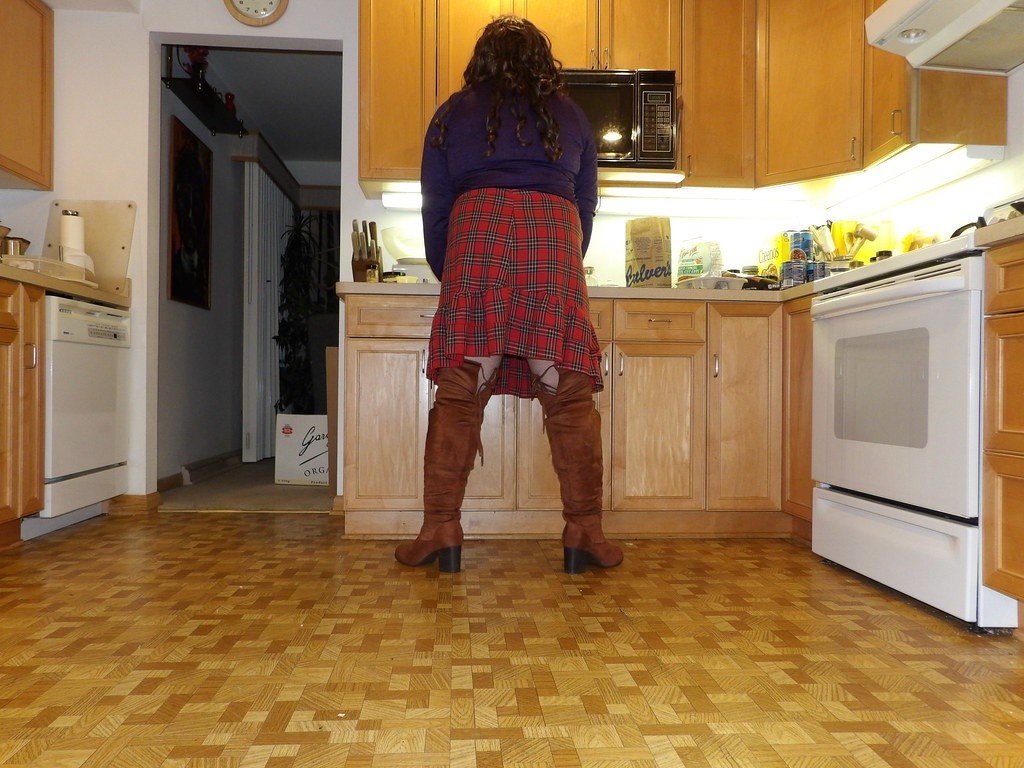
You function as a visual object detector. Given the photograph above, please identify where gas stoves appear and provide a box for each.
[814,189,1024,296]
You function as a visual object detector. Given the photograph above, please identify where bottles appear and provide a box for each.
[742,266,759,275]
[869,247,892,263]
[365,264,379,282]
[728,269,740,274]
[383,271,406,283]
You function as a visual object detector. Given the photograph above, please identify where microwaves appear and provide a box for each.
[555,69,678,168]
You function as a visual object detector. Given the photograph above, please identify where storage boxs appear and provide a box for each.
[275,413,330,486]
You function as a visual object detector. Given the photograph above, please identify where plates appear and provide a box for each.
[675,277,747,290]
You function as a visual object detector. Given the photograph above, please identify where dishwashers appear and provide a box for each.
[19,296,132,539]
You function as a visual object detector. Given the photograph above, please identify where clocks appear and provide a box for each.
[224,0,289,27]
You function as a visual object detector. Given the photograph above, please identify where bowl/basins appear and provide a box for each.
[381,223,428,265]
[394,276,419,283]
[0,221,11,245]
[1,236,31,255]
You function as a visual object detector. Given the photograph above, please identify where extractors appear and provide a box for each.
[864,0,1024,76]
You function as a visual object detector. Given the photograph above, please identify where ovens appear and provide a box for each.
[810,256,1017,631]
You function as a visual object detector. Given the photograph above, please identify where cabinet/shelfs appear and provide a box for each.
[357,0,1007,201]
[344,294,819,539]
[0,0,54,191]
[0,276,46,551]
[982,238,1024,599]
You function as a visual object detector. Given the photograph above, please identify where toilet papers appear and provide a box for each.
[61,209,96,277]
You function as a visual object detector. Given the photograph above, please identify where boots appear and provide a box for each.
[395,359,499,573]
[531,362,624,575]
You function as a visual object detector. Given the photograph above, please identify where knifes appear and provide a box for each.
[352,220,378,261]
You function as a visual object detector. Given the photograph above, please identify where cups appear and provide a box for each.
[7,240,20,256]
[830,261,850,277]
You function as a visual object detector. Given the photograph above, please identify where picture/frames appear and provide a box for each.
[166,115,214,310]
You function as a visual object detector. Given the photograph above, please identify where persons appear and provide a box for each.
[393,18,624,571]
